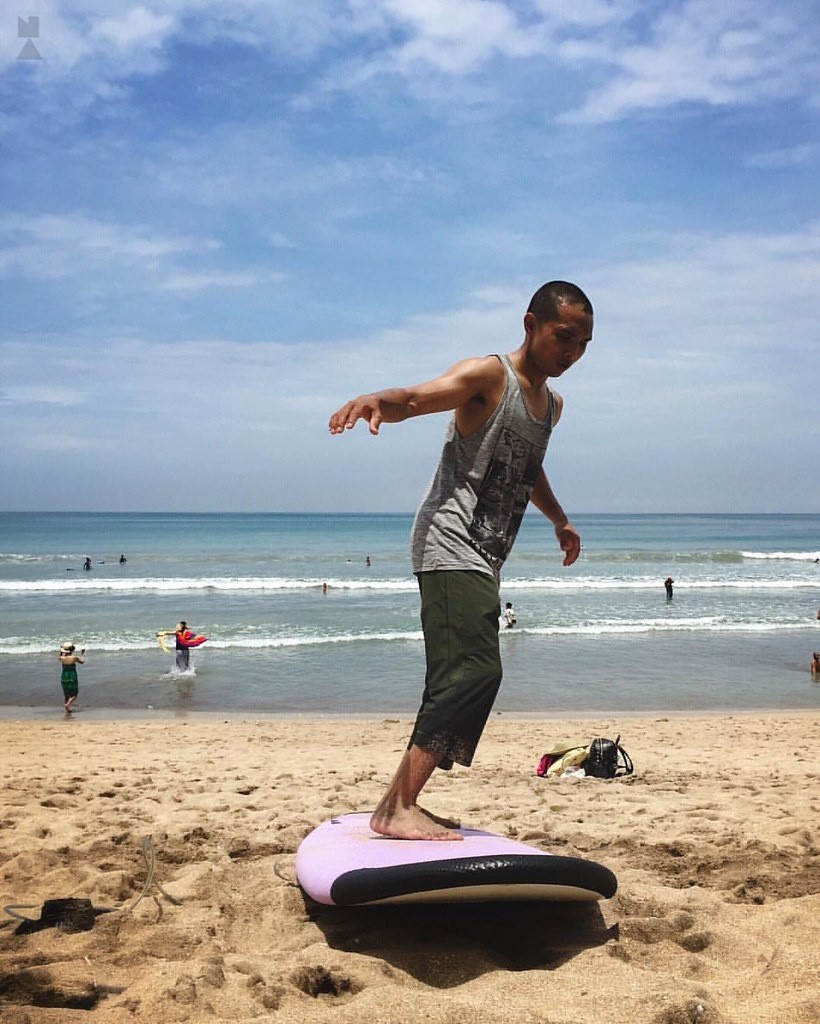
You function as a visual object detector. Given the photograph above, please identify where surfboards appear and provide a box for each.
[295,811,618,908]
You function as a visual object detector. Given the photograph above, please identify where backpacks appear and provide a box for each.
[581,735,633,779]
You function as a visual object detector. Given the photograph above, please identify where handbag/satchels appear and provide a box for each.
[536,754,561,777]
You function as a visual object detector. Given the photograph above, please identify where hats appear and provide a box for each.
[545,739,589,755]
[61,642,75,653]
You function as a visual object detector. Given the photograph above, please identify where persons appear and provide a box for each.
[323,555,371,594]
[156,621,206,672]
[58,641,85,713]
[665,577,674,600]
[329,280,596,840]
[502,603,515,629]
[83,558,91,570]
[809,610,820,676]
[119,555,128,563]
